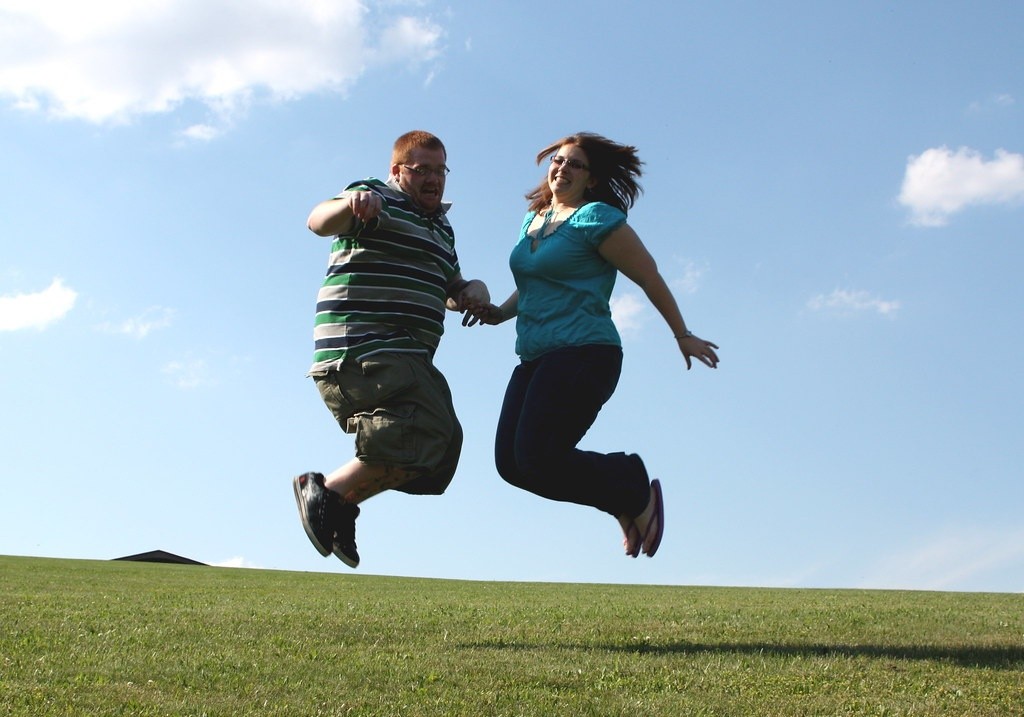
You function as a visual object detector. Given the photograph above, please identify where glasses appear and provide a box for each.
[550,156,594,176]
[396,162,450,177]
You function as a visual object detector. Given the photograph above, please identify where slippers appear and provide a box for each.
[641,479,664,558]
[624,519,642,559]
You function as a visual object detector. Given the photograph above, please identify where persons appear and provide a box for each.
[467,132,719,558]
[293,130,490,568]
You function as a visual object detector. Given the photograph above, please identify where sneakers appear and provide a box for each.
[331,504,360,568]
[292,472,346,557]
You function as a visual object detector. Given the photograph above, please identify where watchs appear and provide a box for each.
[674,331,691,339]
